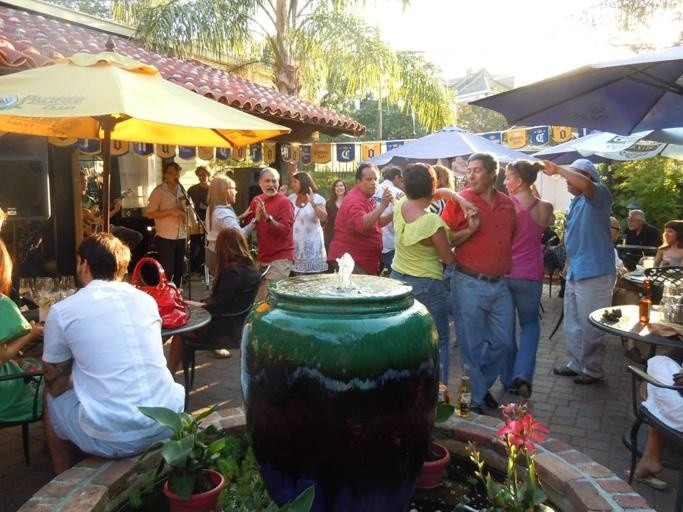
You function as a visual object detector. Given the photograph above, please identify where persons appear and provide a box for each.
[0,238,49,424]
[205,174,265,358]
[42,233,186,477]
[79,172,142,252]
[146,162,213,288]
[387,154,616,413]
[167,227,261,378]
[238,163,405,302]
[605,204,682,359]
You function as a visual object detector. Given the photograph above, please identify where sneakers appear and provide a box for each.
[554,366,578,376]
[483,390,500,410]
[471,406,485,415]
[515,377,532,399]
[573,371,607,385]
[624,347,648,364]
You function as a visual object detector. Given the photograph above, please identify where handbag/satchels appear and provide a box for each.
[128,257,192,330]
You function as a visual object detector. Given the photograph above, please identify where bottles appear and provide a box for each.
[639,278,652,324]
[456,374,472,417]
[660,280,683,324]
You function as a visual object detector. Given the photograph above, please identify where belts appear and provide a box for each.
[454,264,501,285]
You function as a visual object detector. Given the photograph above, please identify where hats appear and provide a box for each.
[569,157,600,185]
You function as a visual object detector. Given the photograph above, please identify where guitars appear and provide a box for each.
[82,187,134,237]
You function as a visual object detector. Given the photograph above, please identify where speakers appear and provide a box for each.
[234,168,263,210]
[0,133,52,221]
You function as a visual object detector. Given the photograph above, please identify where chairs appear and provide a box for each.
[644,265,683,305]
[0,369,45,468]
[538,235,557,319]
[616,246,645,271]
[181,263,273,412]
[549,270,567,340]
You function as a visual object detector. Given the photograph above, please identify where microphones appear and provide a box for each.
[174,178,186,192]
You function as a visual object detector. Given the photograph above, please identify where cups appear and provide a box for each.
[17,275,76,309]
[618,234,627,248]
[639,252,682,273]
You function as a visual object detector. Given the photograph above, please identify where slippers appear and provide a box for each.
[624,468,668,490]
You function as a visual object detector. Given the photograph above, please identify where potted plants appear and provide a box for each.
[414,403,455,487]
[136,401,225,512]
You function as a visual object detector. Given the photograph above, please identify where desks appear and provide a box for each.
[620,270,644,293]
[161,300,212,414]
[586,304,683,359]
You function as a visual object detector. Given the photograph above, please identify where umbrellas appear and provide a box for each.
[0,38,291,233]
